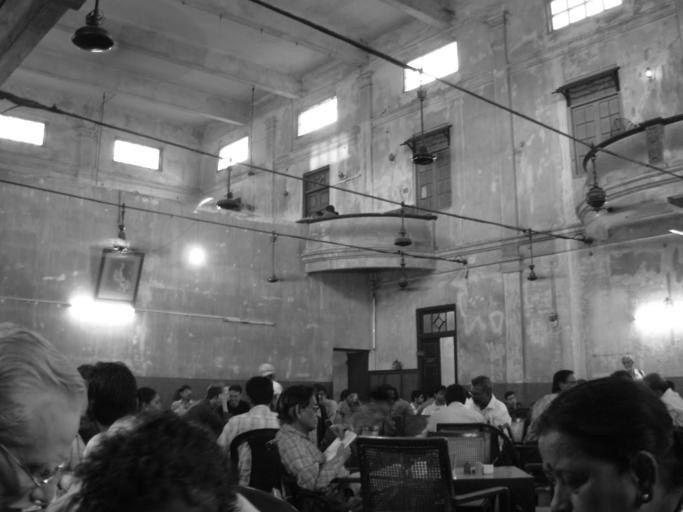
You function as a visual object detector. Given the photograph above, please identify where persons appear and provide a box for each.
[524,369,682,511]
[622,355,646,382]
[0,320,90,511]
[642,373,682,429]
[530,366,577,428]
[31,360,529,511]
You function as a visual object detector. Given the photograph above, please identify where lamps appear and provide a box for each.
[397,252,408,288]
[584,142,607,212]
[524,228,540,281]
[70,0,114,54]
[393,198,413,247]
[111,201,132,255]
[266,229,280,283]
[215,164,244,214]
[411,84,435,165]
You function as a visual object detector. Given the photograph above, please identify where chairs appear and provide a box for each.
[228,405,554,512]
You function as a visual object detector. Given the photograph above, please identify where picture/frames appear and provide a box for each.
[93,247,145,303]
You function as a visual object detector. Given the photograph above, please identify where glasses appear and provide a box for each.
[301,403,319,413]
[0,443,66,492]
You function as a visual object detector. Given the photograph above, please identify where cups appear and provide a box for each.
[480,463,493,475]
[447,451,457,479]
